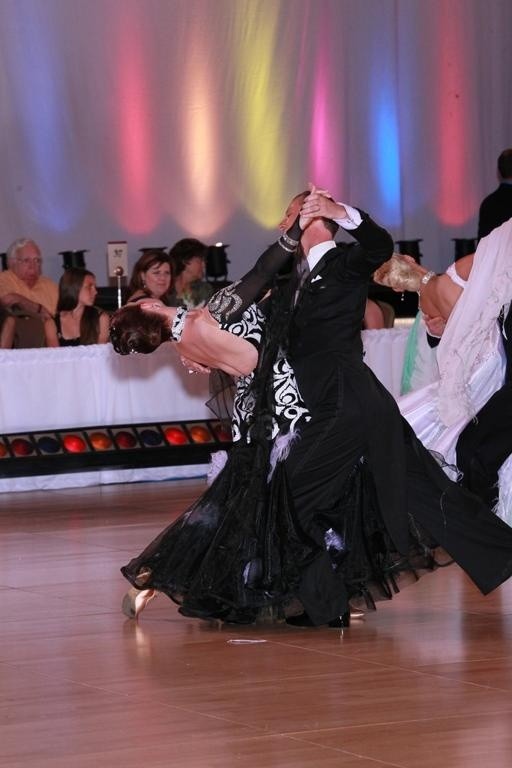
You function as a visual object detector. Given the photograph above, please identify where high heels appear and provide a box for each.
[116,560,159,625]
[348,600,367,622]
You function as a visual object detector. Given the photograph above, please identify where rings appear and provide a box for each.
[188,369,197,376]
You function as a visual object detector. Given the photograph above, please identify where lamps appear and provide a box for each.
[449,237,480,265]
[137,246,168,254]
[57,248,89,272]
[394,238,424,266]
[205,241,232,282]
[0,252,9,272]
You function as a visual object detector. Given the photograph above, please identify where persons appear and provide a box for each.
[364,217,512,526]
[476,148,512,237]
[0,238,209,349]
[105,183,367,623]
[176,184,511,631]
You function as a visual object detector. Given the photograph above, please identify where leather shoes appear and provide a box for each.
[285,600,353,634]
